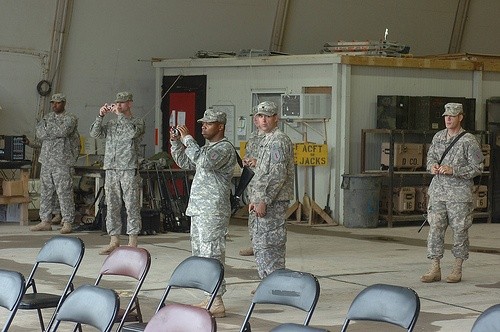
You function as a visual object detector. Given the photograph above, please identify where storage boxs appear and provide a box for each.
[380,142,491,214]
[3,181,24,197]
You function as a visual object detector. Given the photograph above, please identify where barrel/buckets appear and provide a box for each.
[341,175,383,228]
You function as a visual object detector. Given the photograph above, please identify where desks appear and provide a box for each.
[0,159,32,226]
[67,166,195,230]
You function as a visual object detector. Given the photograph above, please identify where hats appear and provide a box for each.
[441,102,463,116]
[250,105,257,116]
[255,101,277,117]
[197,109,226,125]
[113,92,133,102]
[49,94,66,103]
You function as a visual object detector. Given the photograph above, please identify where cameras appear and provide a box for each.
[173,129,180,136]
[105,106,113,112]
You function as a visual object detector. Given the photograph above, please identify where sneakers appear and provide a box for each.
[51,214,61,224]
[30,221,52,231]
[60,222,72,234]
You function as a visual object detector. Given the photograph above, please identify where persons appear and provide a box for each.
[170,109,237,317]
[239,102,294,297]
[90,92,146,255]
[419,103,484,282]
[22,93,81,234]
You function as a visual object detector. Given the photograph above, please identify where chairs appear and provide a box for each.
[0,236,500,332]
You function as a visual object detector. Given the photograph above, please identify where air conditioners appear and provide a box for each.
[281,94,331,118]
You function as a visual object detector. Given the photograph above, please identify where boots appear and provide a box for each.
[420,259,442,282]
[446,258,463,282]
[194,295,225,317]
[100,235,119,255]
[239,239,253,256]
[129,234,138,247]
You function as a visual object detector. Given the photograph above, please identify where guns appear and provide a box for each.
[230,164,257,212]
[91,163,191,234]
[418,212,430,233]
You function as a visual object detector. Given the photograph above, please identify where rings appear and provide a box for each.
[440,170,441,172]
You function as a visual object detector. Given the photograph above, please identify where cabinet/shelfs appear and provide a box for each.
[361,128,494,229]
